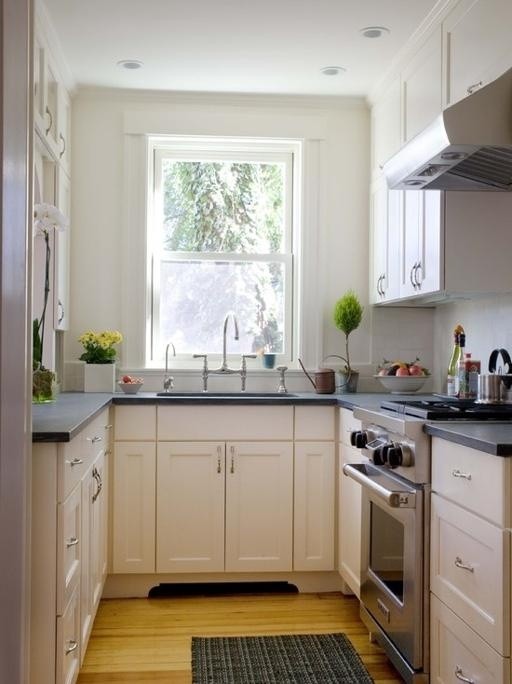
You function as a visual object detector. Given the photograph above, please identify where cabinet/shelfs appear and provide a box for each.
[419,419,511,683]
[370,22,512,308]
[105,396,337,603]
[0,1,76,378]
[22,396,113,682]
[337,393,366,605]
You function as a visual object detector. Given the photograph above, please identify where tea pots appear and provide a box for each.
[476,348,512,404]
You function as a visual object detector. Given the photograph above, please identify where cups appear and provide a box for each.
[262,353,276,369]
[312,368,336,395]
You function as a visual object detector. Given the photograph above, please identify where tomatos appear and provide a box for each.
[123,376,131,383]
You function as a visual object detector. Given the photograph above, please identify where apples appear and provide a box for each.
[379,369,388,376]
[396,365,431,376]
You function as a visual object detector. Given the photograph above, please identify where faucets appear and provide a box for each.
[165,343,176,376]
[221,310,240,366]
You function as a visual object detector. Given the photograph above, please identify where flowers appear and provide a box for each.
[30,199,73,368]
[75,328,125,363]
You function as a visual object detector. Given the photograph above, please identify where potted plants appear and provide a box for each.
[332,286,365,396]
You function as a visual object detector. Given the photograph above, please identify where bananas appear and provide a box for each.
[392,362,407,367]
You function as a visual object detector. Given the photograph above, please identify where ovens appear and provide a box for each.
[342,462,433,684]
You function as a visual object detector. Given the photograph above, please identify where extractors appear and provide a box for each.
[381,66,512,192]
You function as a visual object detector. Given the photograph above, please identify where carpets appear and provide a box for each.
[187,630,377,683]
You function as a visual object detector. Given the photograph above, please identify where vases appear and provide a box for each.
[82,361,118,395]
[30,364,58,404]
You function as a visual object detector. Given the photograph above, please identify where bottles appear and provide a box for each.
[446,324,467,398]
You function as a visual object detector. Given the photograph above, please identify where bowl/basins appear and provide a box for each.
[119,382,144,395]
[374,373,431,397]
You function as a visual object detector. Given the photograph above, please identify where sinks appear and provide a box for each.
[158,392,300,399]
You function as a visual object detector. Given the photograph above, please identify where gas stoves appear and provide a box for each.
[348,401,512,485]
